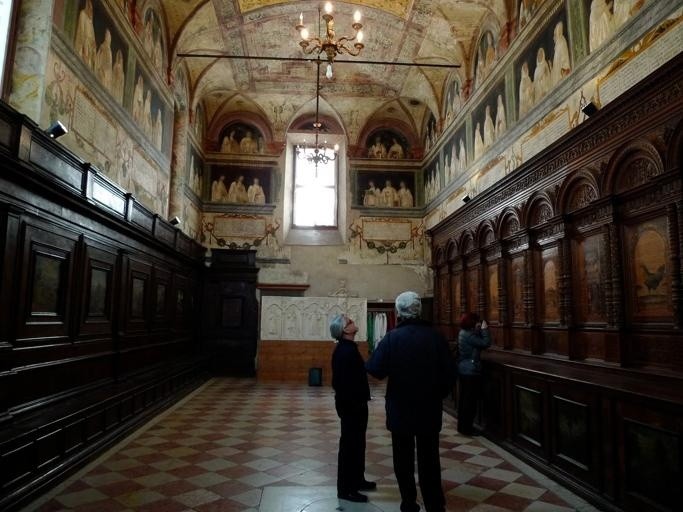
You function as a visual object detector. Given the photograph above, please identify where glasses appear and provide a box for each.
[345,320,352,328]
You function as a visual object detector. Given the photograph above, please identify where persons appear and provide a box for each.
[363,134,414,209]
[456,312,492,438]
[73,0,163,154]
[364,290,458,512]
[329,315,376,503]
[211,130,266,204]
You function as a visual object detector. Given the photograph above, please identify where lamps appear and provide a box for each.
[294,60,339,177]
[295,1,364,64]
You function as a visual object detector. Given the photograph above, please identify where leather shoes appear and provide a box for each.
[337,491,368,503]
[360,480,377,491]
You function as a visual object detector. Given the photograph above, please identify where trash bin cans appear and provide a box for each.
[309,368,322,386]
